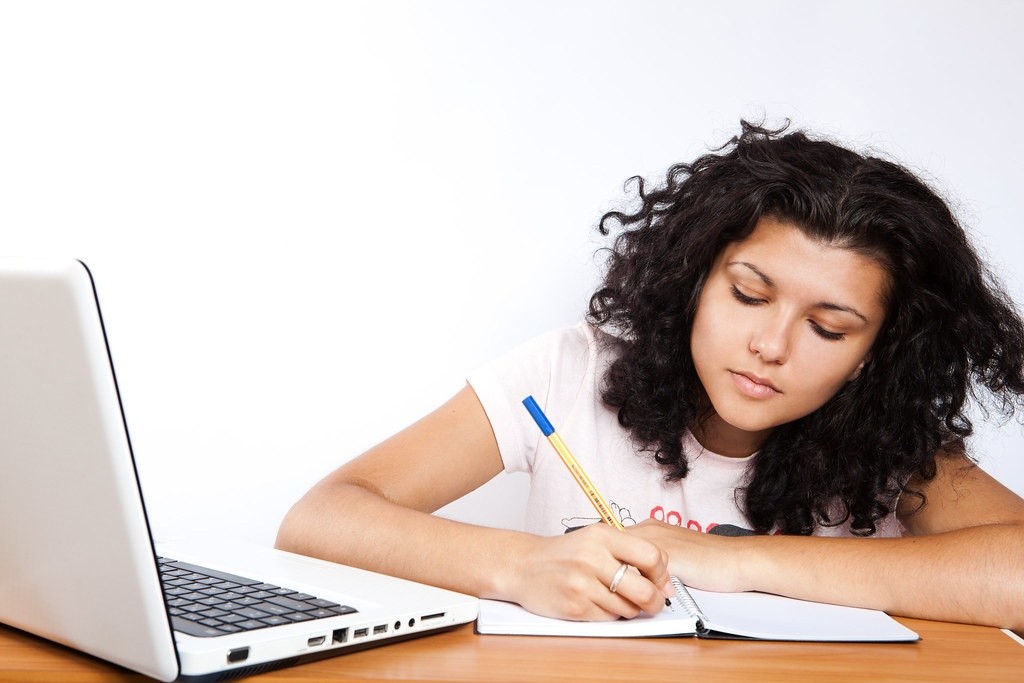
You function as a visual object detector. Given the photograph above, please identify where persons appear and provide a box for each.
[273,116,1024,634]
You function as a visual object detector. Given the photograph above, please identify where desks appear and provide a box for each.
[0,613,1024,683]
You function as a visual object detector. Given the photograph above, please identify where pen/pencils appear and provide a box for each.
[521,394,675,612]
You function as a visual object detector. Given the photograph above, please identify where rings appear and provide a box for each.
[608,563,629,593]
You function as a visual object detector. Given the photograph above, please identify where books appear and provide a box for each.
[475,575,923,642]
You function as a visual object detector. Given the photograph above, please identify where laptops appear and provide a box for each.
[0,259,479,683]
